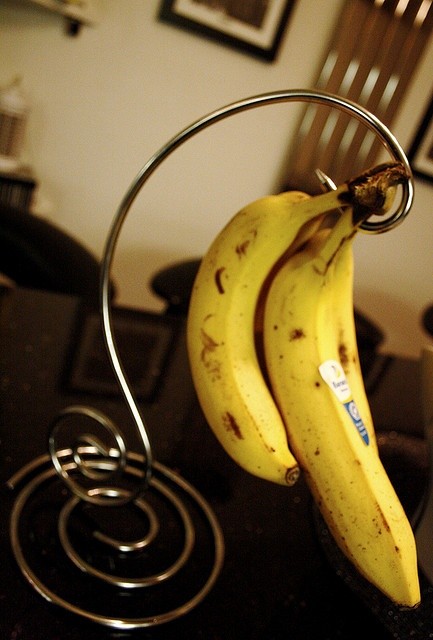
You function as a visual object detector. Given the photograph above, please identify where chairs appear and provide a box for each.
[146,253,387,360]
[0,204,116,304]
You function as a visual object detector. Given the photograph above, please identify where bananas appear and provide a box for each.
[185,160,423,612]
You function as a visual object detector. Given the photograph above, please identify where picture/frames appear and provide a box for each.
[57,298,181,411]
[154,0,299,65]
